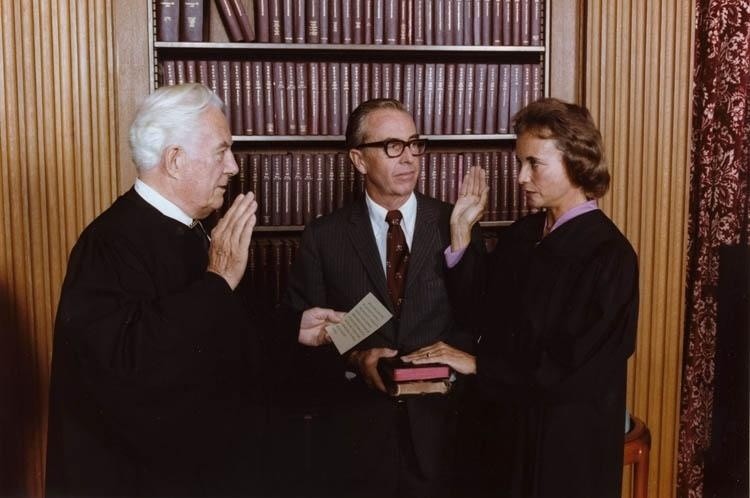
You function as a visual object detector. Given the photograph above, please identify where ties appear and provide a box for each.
[385,210,410,316]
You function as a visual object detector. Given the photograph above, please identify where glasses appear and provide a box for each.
[359,140,428,157]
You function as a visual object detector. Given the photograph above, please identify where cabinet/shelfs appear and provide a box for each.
[115,0,587,323]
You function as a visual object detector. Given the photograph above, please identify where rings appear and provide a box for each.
[427,352,430,357]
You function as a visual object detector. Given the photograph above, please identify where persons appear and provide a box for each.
[42,83,347,498]
[290,99,487,498]
[400,97,639,497]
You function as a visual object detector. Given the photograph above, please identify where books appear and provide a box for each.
[378,357,452,398]
[418,151,540,221]
[157,61,540,137]
[156,2,541,47]
[210,153,365,226]
[244,235,302,308]
